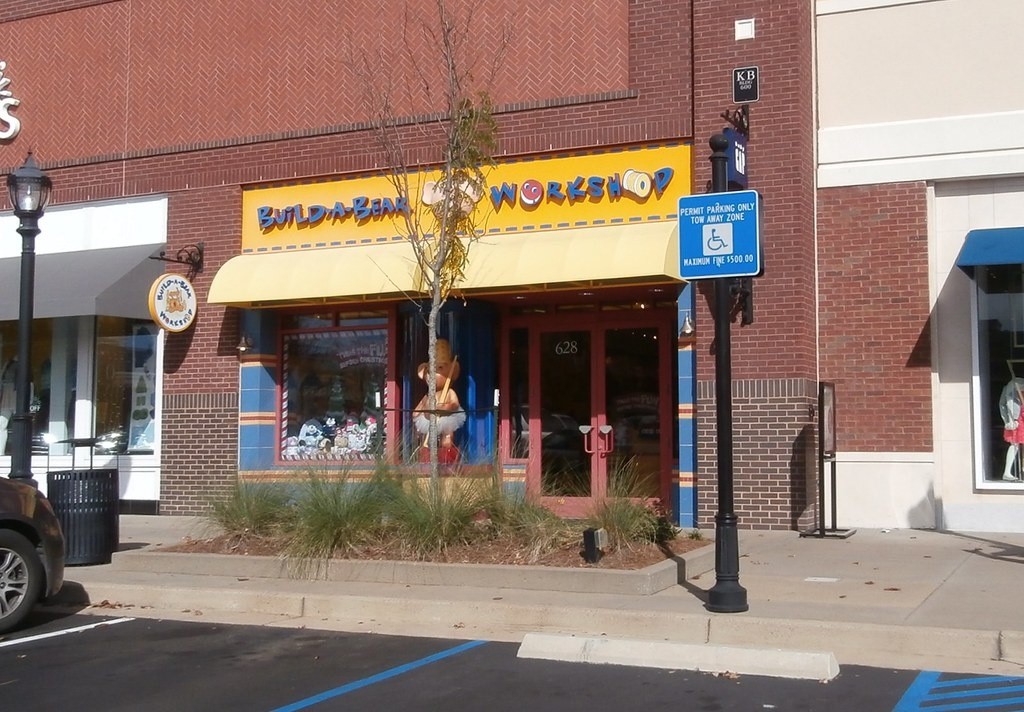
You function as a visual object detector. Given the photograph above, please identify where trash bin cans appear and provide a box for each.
[45,435,121,567]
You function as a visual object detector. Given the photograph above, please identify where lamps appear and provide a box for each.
[679,310,696,334]
[235,334,252,351]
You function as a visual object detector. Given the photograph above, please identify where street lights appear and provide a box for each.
[4,147,54,484]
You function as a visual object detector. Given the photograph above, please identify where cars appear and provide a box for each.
[511,403,585,477]
[1,475,67,636]
